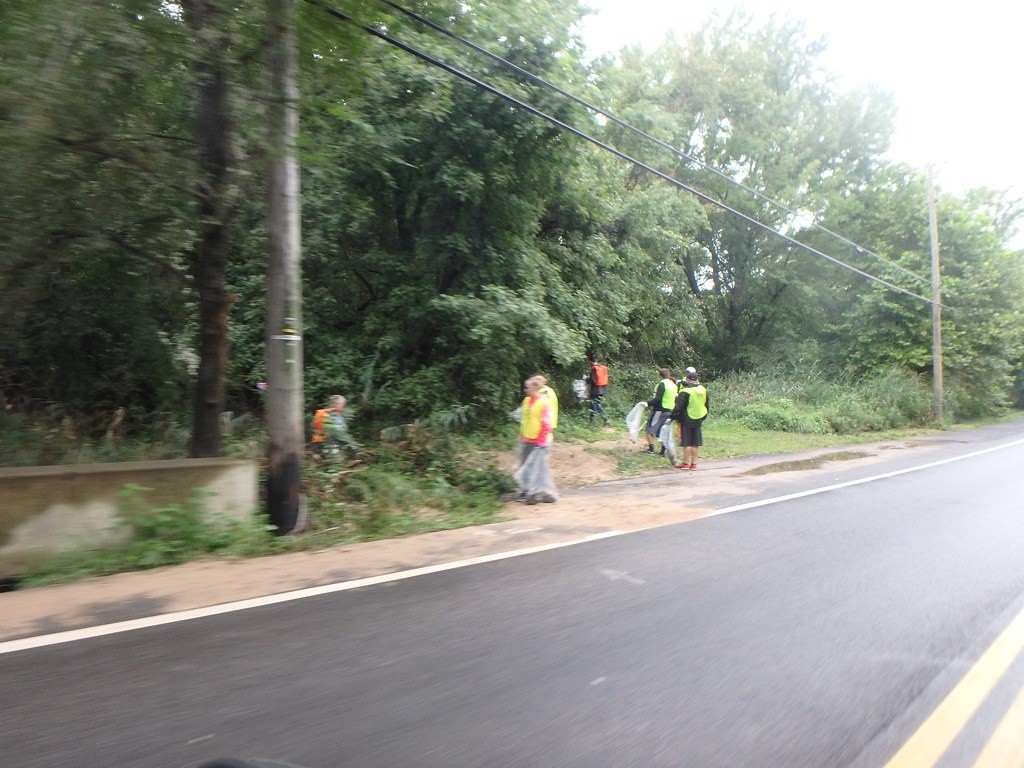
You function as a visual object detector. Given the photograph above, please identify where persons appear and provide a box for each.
[311,395,359,494]
[676,366,697,393]
[584,359,611,426]
[666,372,709,470]
[517,374,557,505]
[641,368,679,457]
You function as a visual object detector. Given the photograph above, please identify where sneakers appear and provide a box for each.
[673,463,690,469]
[690,464,697,470]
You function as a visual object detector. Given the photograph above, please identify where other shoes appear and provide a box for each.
[639,449,655,456]
[516,496,526,502]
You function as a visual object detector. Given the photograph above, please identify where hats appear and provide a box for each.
[687,373,698,381]
[686,367,696,373]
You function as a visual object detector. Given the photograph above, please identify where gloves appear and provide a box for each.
[666,419,672,425]
[640,402,648,407]
[583,374,587,381]
[676,379,682,385]
